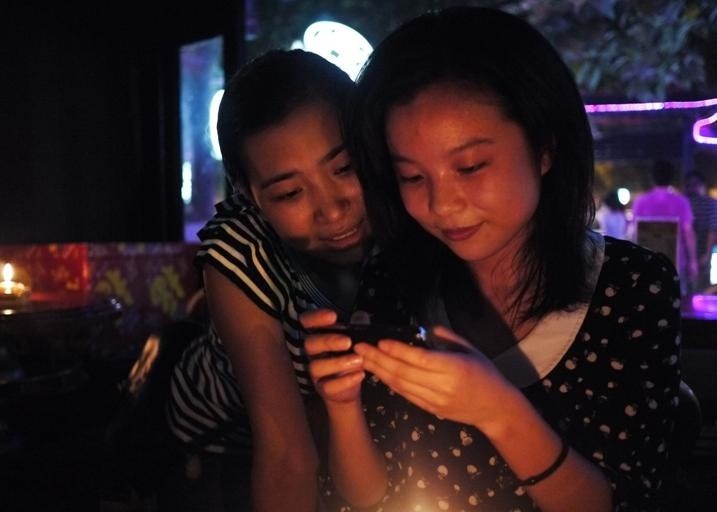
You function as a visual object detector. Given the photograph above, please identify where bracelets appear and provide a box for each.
[513,430,570,487]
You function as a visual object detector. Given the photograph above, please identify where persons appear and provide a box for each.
[301,3,688,509]
[156,44,368,510]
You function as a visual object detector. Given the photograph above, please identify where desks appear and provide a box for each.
[0,292,122,336]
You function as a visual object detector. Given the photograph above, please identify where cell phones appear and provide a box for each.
[322,322,429,357]
